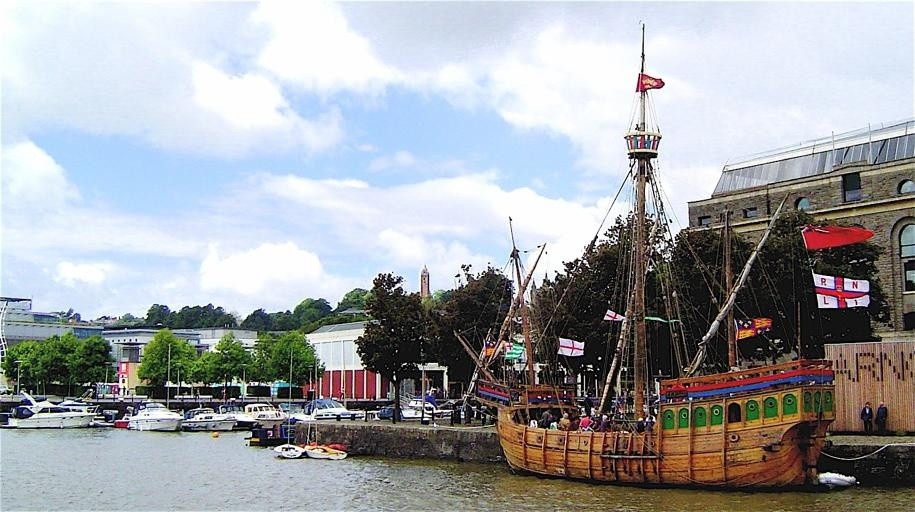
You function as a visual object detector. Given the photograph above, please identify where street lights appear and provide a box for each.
[14,361,23,395]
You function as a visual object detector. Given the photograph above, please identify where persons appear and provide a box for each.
[861,402,873,436]
[528,392,654,432]
[876,401,887,435]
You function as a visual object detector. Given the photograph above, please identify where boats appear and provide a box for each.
[400,398,453,421]
[0,390,102,429]
[121,399,184,432]
[817,472,856,489]
[182,396,350,431]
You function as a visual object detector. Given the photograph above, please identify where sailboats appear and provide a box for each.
[306,359,348,461]
[272,351,306,459]
[453,27,836,488]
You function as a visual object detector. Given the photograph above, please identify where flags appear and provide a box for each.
[737,317,772,339]
[636,74,665,92]
[558,338,584,357]
[604,309,623,322]
[803,224,874,250]
[814,273,870,309]
[486,340,497,356]
[499,342,524,358]
